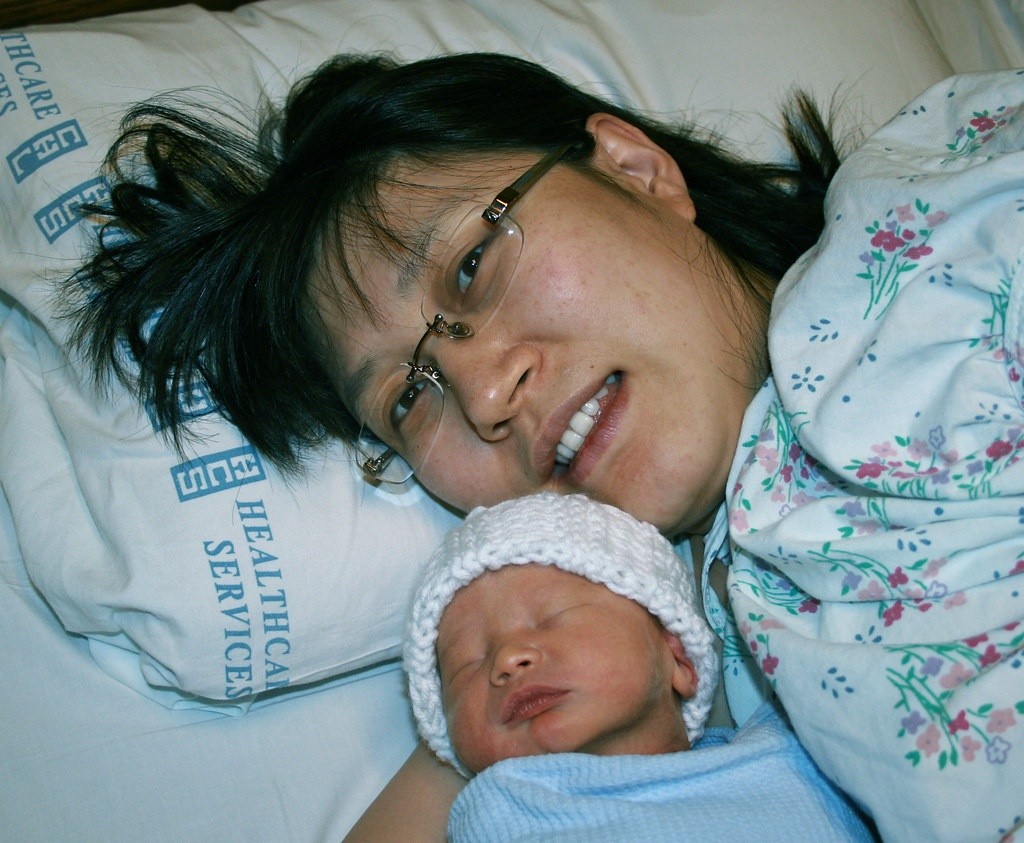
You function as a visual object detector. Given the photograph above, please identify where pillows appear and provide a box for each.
[0,0,1014,717]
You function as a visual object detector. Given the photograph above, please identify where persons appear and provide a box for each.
[56,48,1024,843]
[402,494,872,843]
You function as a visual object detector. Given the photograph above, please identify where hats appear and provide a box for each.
[402,492,720,780]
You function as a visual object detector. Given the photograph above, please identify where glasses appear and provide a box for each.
[356,142,576,488]
